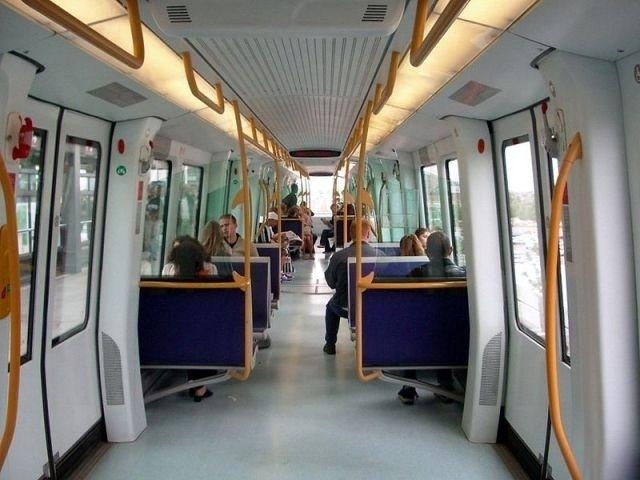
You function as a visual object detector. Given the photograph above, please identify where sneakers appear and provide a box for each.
[398,386,416,404]
[317,245,332,254]
[434,393,455,408]
[281,273,294,281]
[323,344,335,354]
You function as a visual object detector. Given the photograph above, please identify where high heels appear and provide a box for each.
[193,389,213,402]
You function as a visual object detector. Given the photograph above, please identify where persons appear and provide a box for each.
[254,211,295,282]
[399,228,467,404]
[282,184,317,253]
[322,218,388,354]
[318,203,356,254]
[162,214,259,405]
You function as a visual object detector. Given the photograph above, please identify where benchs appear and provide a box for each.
[139,218,303,403]
[333,217,467,403]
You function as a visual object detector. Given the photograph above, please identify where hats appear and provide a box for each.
[268,212,278,221]
